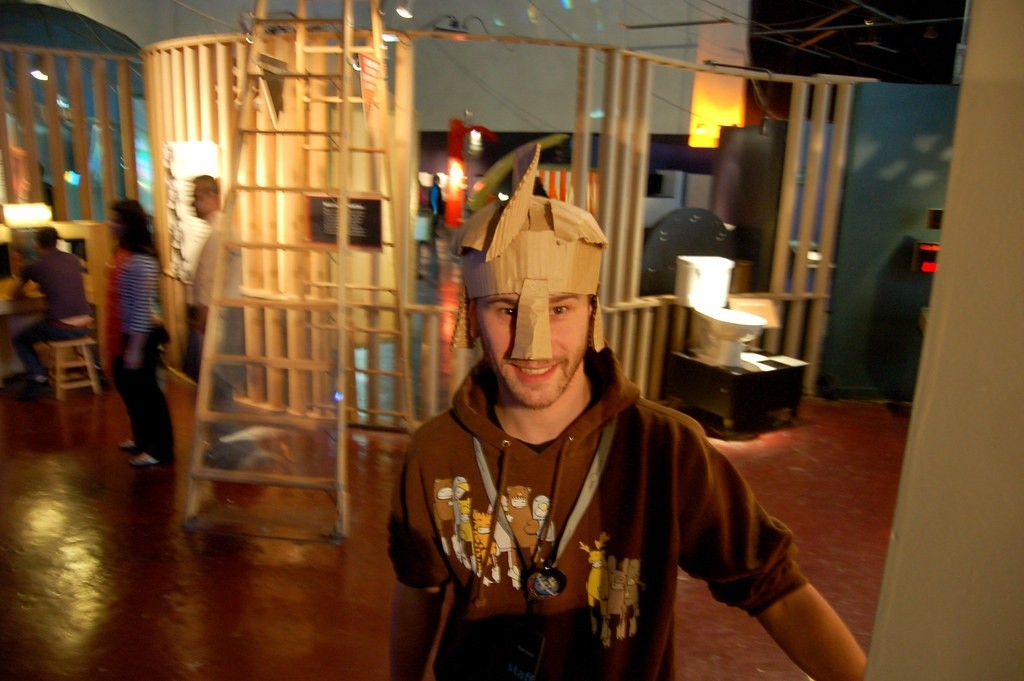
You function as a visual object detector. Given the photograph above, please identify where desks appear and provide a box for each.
[664,346,806,434]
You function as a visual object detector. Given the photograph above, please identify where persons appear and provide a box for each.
[107,200,175,465]
[385,195,865,681]
[186,175,234,406]
[429,176,442,237]
[8,228,93,390]
[40,164,53,204]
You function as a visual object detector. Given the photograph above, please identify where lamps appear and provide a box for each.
[395,0,414,19]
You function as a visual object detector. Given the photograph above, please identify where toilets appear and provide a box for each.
[673,256,767,367]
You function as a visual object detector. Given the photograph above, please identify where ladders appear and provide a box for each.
[181,0,419,540]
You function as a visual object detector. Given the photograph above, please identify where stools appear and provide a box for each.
[47,336,100,402]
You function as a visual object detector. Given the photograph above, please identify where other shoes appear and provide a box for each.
[16,379,52,400]
[118,439,137,450]
[128,452,173,466]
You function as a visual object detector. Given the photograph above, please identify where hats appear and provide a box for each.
[450,144,605,360]
